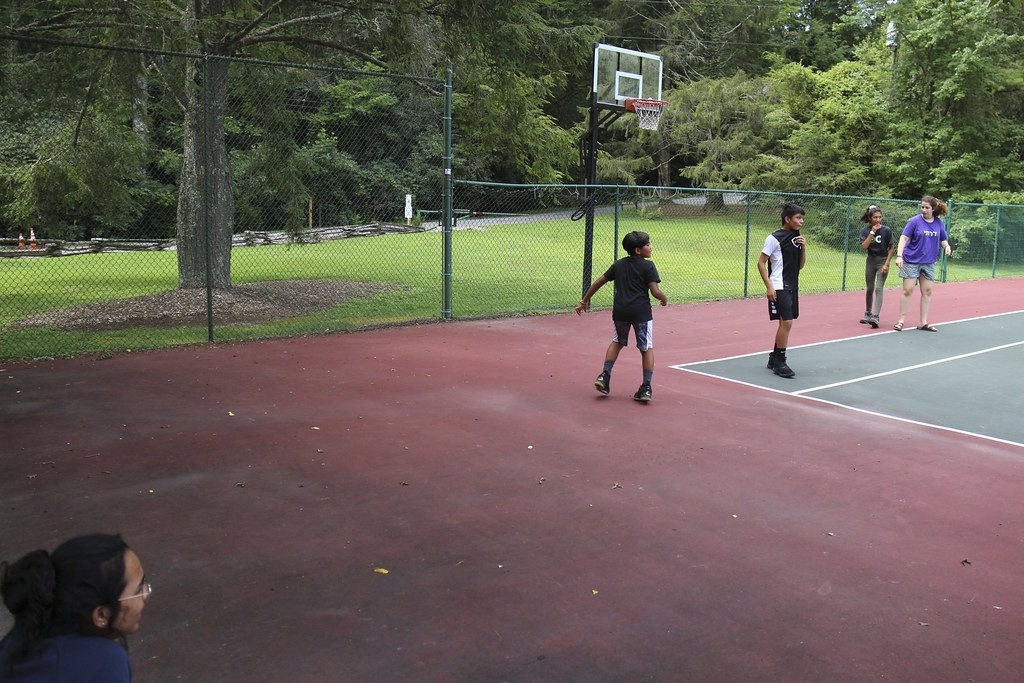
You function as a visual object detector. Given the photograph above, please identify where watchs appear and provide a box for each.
[868,231,877,235]
[856,202,894,330]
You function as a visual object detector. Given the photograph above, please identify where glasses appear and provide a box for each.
[118,581,152,605]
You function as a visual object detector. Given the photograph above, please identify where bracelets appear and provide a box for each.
[869,234,873,236]
[896,254,904,258]
[944,244,949,249]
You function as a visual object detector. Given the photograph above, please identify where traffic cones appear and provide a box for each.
[18,232,26,250]
[29,227,37,247]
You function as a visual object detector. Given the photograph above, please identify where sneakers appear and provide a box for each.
[868,315,880,328]
[767,352,774,369]
[634,386,652,401]
[860,311,872,323]
[773,352,795,376]
[595,375,610,394]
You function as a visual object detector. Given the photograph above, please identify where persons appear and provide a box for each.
[574,229,667,405]
[893,196,954,333]
[756,200,806,378]
[1,534,158,683]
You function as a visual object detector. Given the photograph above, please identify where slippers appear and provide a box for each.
[893,322,903,331]
[917,323,937,331]
